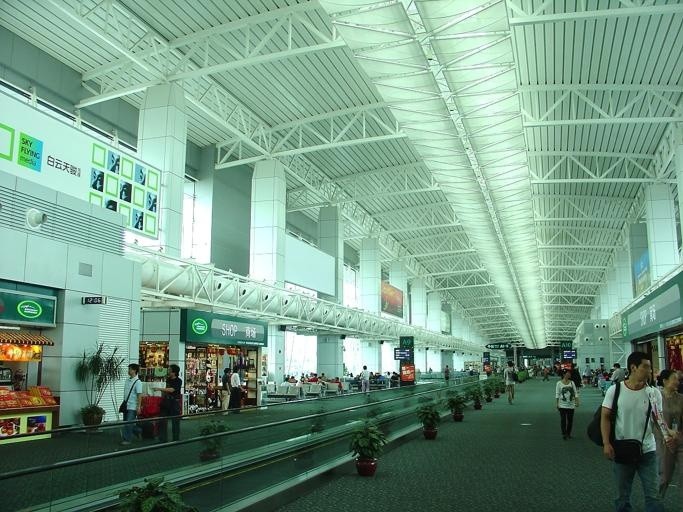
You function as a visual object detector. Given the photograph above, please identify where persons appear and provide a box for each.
[120,362,143,446]
[656,369,682,498]
[467,362,625,396]
[663,431,682,449]
[151,362,182,444]
[444,364,450,385]
[230,365,246,415]
[219,367,231,417]
[503,359,517,406]
[281,371,400,395]
[358,365,372,394]
[599,349,667,511]
[554,368,580,440]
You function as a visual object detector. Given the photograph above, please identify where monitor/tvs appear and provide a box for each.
[482,358,489,362]
[394,348,411,360]
[564,350,576,358]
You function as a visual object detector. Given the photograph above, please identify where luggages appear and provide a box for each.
[140,420,159,439]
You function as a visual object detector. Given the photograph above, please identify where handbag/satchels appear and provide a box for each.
[118,400,126,413]
[587,379,621,446]
[613,438,643,464]
[512,372,518,382]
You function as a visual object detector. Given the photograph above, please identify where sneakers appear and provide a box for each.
[118,439,133,445]
[562,435,572,440]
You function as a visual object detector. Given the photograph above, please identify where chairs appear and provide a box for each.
[264,374,400,400]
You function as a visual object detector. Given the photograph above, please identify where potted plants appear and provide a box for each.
[309,407,326,432]
[74,342,125,429]
[447,381,506,423]
[199,422,231,461]
[415,406,439,440]
[346,418,388,476]
